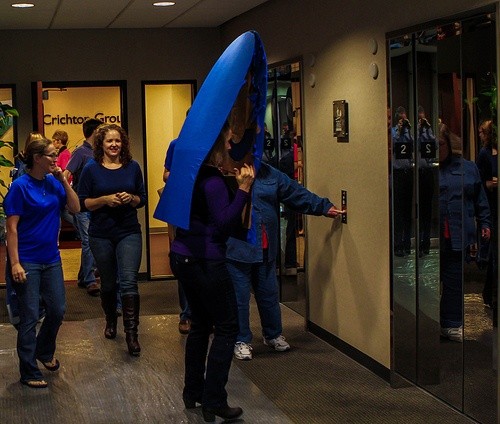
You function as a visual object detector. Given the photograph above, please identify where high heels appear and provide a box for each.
[201,400,243,422]
[182,387,203,409]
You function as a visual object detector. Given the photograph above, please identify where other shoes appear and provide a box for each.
[179,320,191,333]
[37,316,45,323]
[87,284,100,294]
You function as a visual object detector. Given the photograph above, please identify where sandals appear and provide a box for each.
[20,378,48,387]
[38,357,60,372]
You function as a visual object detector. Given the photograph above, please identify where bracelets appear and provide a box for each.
[130,193,135,204]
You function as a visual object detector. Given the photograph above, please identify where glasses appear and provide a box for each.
[228,158,254,167]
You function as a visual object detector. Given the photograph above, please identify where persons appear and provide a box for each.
[439,123,491,343]
[2,117,145,388]
[416,105,438,258]
[474,120,498,330]
[387,108,392,204]
[279,122,291,154]
[280,152,300,276]
[392,105,413,256]
[261,121,275,164]
[152,105,345,423]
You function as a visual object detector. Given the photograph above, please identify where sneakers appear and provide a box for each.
[234,342,253,360]
[264,335,290,352]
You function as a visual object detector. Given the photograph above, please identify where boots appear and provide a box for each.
[120,294,140,352]
[100,288,118,338]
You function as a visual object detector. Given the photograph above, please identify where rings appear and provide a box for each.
[15,276,19,280]
[112,202,116,206]
